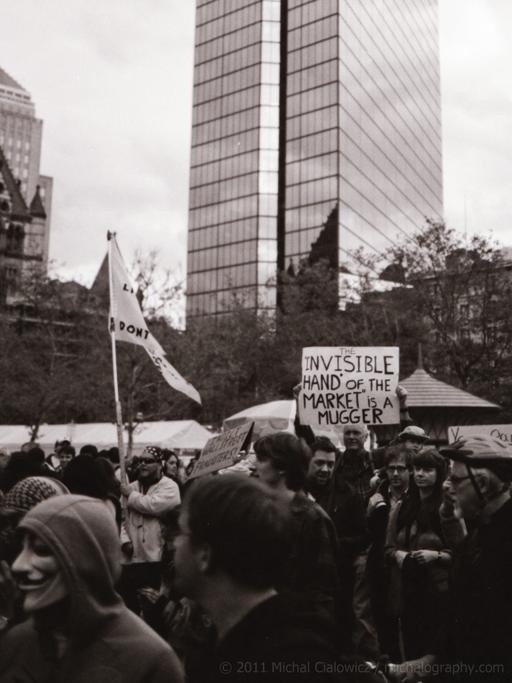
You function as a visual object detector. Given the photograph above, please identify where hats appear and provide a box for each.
[398,426,430,439]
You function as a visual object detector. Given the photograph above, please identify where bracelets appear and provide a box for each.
[400,407,410,412]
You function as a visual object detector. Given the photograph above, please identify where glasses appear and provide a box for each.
[137,458,156,464]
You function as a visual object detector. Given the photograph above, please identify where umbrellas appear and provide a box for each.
[222,399,368,444]
[398,366,500,420]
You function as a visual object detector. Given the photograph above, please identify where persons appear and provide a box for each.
[292,381,414,507]
[0,432,512,681]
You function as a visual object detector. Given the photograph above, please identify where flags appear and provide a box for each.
[109,235,203,409]
